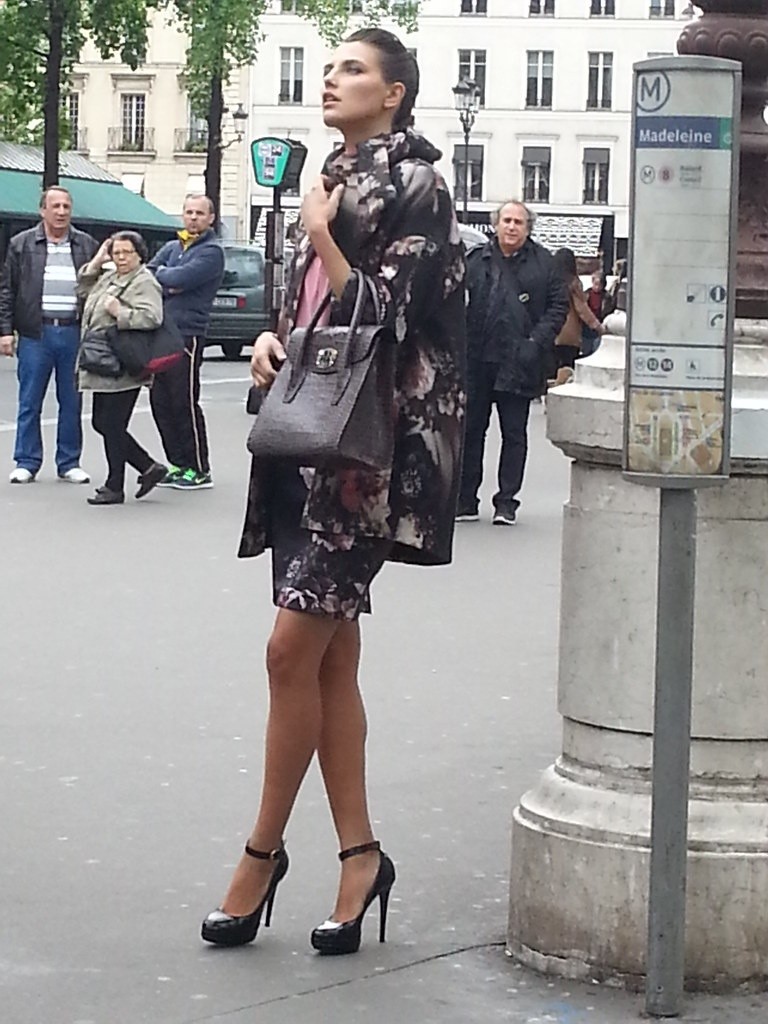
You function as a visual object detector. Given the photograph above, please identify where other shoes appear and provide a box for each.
[65,468,91,484]
[137,465,169,498]
[87,486,126,505]
[10,467,36,484]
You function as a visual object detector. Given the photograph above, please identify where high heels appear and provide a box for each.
[310,840,396,955]
[201,839,289,948]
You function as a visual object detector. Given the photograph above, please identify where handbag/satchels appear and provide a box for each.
[106,304,184,382]
[80,329,124,377]
[245,271,399,474]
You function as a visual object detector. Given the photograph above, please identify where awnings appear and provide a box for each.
[0,173,183,230]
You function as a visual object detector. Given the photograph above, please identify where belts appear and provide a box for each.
[43,317,78,326]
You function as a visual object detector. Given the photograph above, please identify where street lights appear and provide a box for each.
[217,103,249,234]
[452,69,481,223]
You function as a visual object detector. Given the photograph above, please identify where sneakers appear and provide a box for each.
[493,505,517,525]
[455,498,480,521]
[156,465,215,490]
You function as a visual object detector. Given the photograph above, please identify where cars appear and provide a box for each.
[202,238,266,358]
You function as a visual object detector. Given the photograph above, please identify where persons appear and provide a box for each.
[0,185,100,484]
[75,231,168,504]
[455,200,570,525]
[145,195,225,490]
[202,29,467,952]
[541,248,627,414]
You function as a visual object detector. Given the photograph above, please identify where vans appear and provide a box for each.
[457,226,491,307]
